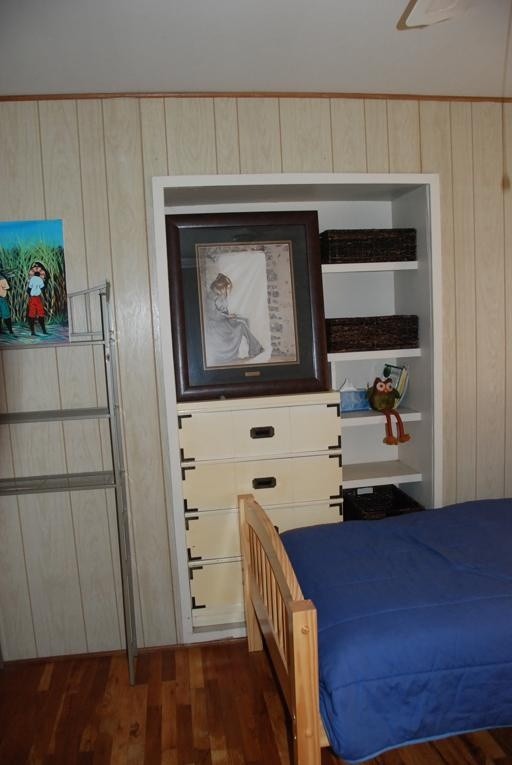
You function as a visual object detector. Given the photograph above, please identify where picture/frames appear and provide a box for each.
[165,210,332,403]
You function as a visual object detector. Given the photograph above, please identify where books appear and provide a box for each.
[381,362,410,410]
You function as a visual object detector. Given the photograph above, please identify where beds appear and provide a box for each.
[238,493,512,765]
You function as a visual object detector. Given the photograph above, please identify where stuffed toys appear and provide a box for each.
[364,377,411,444]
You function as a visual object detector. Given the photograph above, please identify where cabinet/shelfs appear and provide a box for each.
[152,172,444,645]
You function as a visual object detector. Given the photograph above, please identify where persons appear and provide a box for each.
[0,275,18,340]
[26,272,50,336]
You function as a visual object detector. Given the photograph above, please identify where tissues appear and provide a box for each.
[340,377,372,413]
